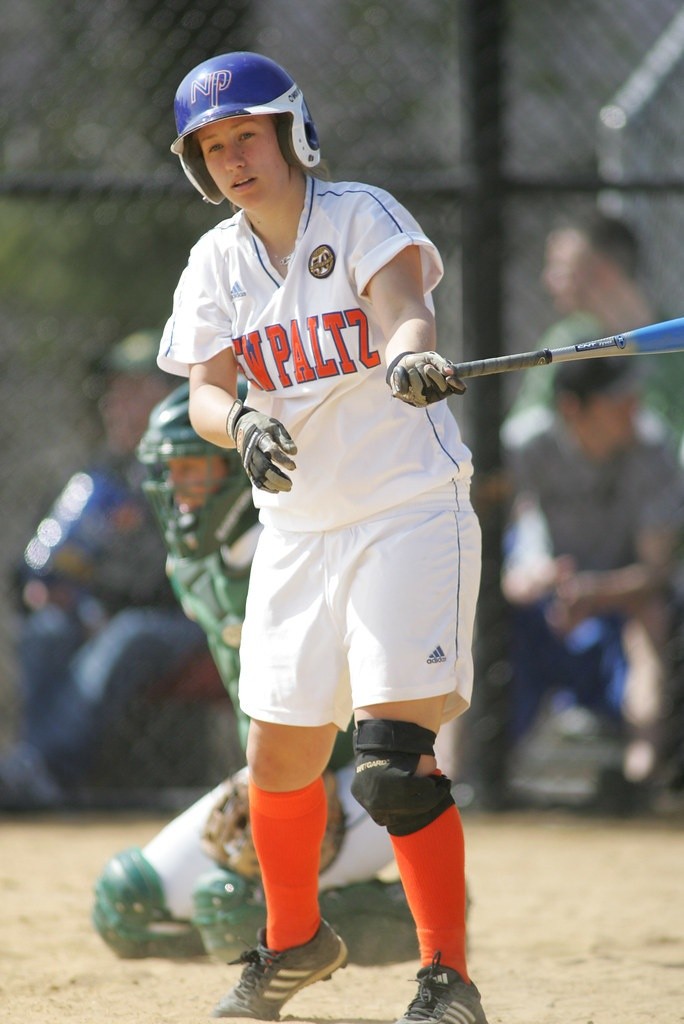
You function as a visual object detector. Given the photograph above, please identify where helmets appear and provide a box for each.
[170,52,320,205]
[140,380,260,562]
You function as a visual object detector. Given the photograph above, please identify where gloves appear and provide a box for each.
[226,399,298,493]
[386,351,468,408]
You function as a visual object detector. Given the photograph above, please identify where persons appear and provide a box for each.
[157,52,488,1024]
[0,212,684,816]
[93,373,473,966]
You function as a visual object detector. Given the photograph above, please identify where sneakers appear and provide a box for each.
[212,917,348,1022]
[392,951,489,1024]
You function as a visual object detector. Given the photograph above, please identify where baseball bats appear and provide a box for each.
[452,317,683,380]
